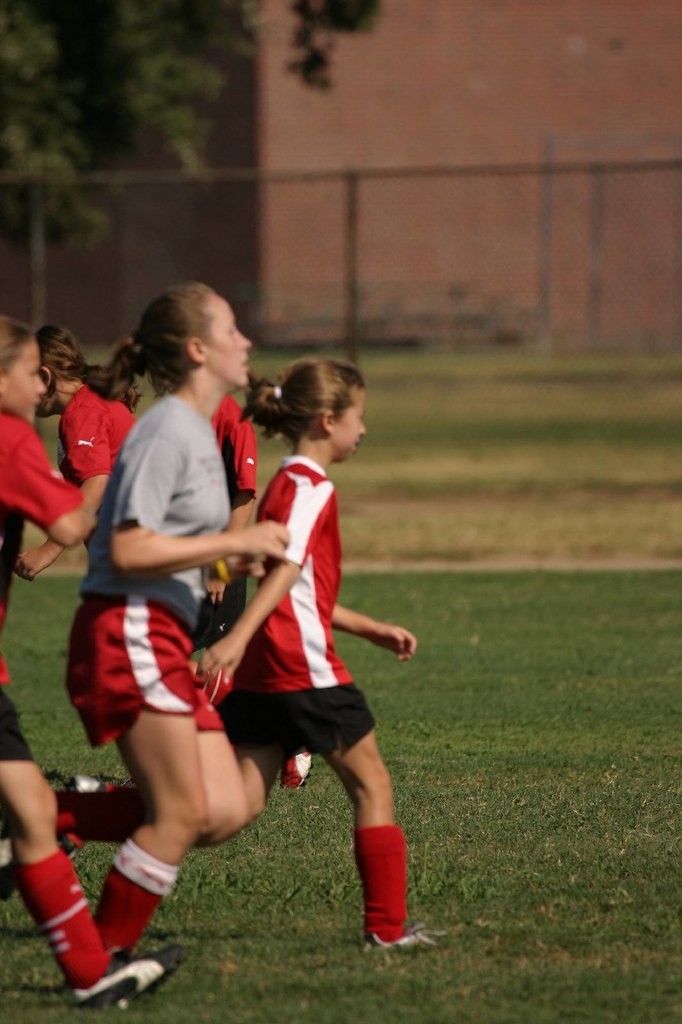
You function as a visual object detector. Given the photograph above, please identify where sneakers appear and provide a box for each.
[362,921,447,952]
[0,799,18,902]
[280,750,314,790]
[56,772,118,859]
[72,943,183,1013]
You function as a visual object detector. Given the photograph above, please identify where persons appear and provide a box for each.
[0,280,292,959]
[1,316,452,1007]
